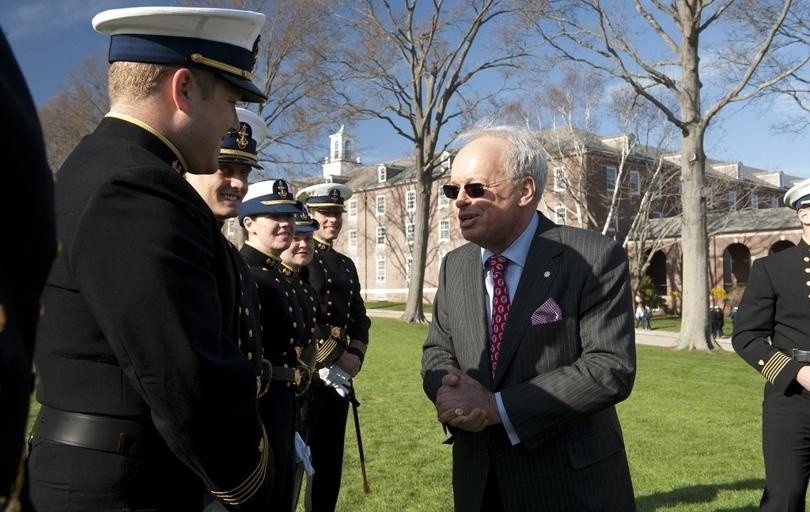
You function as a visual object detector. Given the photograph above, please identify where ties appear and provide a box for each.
[486,256,512,380]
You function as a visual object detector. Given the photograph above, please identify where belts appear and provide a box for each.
[272,366,296,382]
[775,348,809,363]
[32,406,138,458]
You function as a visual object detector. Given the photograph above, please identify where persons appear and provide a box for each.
[182,105,268,226]
[237,179,370,512]
[730,307,739,325]
[22,5,298,512]
[731,179,809,511]
[711,306,724,338]
[420,126,636,512]
[635,297,653,330]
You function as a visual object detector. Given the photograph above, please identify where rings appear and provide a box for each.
[454,407,464,416]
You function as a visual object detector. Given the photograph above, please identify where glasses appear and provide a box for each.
[443,177,517,199]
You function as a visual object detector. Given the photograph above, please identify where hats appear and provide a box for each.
[238,179,301,226]
[295,182,353,214]
[783,177,810,211]
[294,207,320,234]
[91,7,268,104]
[219,108,267,171]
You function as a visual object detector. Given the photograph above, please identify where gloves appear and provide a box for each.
[318,367,351,398]
[325,364,351,388]
